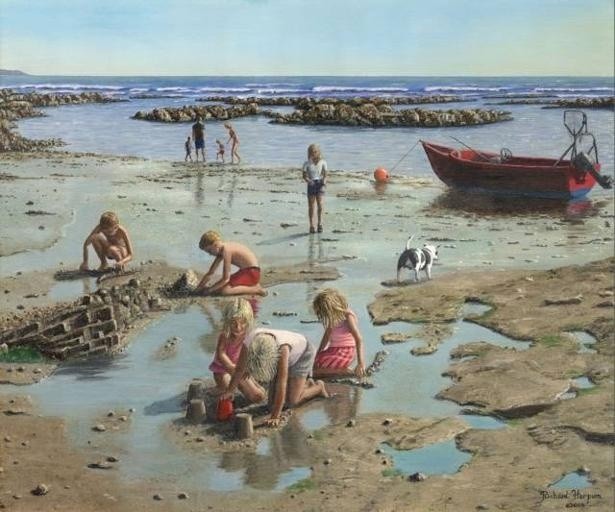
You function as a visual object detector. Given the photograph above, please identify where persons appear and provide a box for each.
[224,122,241,164]
[312,287,366,376]
[302,144,326,233]
[184,137,193,161]
[209,297,266,402]
[216,139,224,161]
[80,211,134,273]
[220,327,330,426]
[196,231,267,296]
[192,115,206,163]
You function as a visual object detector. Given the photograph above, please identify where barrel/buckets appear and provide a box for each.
[374,168,390,183]
[216,397,234,423]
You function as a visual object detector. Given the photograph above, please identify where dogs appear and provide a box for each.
[396,235,440,282]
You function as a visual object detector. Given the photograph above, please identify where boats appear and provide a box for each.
[420,140,601,198]
